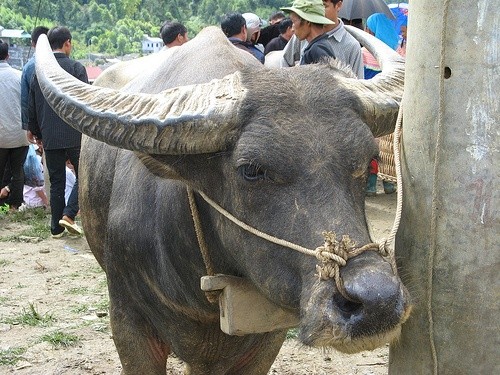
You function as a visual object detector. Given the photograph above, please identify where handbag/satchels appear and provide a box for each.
[23,144,44,187]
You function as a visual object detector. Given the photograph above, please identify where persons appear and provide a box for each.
[279,0,337,65]
[158,19,188,50]
[28,25,89,239]
[0,136,79,213]
[22,26,52,211]
[361,13,406,196]
[281,0,364,80]
[241,11,407,65]
[0,38,30,214]
[221,13,256,60]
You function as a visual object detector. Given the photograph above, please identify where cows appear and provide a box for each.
[35,25,412,374]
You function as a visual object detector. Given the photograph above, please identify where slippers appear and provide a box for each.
[59,220,81,235]
[52,227,68,238]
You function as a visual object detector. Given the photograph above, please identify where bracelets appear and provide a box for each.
[5,186,10,192]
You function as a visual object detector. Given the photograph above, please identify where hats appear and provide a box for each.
[242,13,262,43]
[280,0,335,24]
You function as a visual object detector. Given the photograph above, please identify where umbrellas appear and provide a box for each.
[337,0,397,29]
